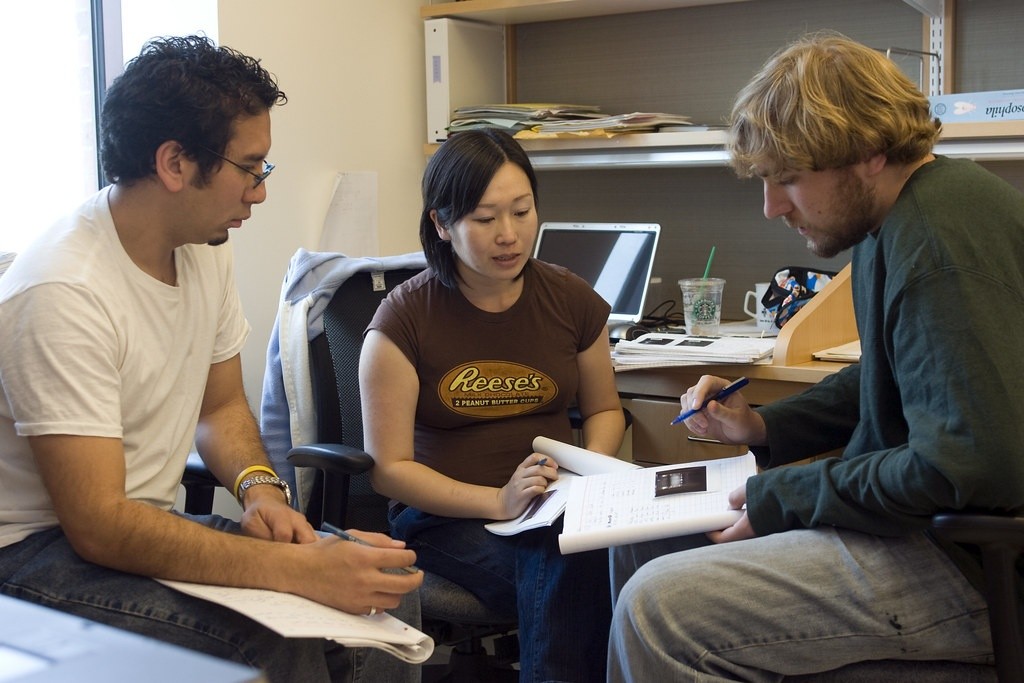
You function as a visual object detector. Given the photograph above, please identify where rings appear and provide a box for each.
[368,606,377,617]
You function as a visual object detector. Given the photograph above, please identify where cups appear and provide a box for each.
[677,277,726,335]
[744,283,778,329]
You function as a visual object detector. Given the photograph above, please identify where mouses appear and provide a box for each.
[609,324,651,343]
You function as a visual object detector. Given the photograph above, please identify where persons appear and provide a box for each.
[0,37,424,683]
[606,31,1024,683]
[357,129,627,683]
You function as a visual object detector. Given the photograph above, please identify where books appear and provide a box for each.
[484,435,645,536]
[811,340,864,365]
[612,333,775,372]
[155,577,435,663]
[450,102,731,133]
[558,451,759,555]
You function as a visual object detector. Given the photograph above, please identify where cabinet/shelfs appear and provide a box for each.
[418,0,1023,468]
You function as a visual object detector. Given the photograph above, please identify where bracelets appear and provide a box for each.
[233,464,277,508]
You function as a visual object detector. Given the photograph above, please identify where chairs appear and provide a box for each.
[827,513,1023,682]
[282,251,520,683]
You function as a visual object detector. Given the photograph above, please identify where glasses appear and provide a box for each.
[197,143,275,189]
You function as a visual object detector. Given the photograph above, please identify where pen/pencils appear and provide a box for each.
[536,458,547,466]
[670,374,750,427]
[318,521,420,577]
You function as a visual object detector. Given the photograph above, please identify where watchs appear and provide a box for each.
[238,475,292,506]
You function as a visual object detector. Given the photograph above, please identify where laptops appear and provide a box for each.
[533,223,661,324]
